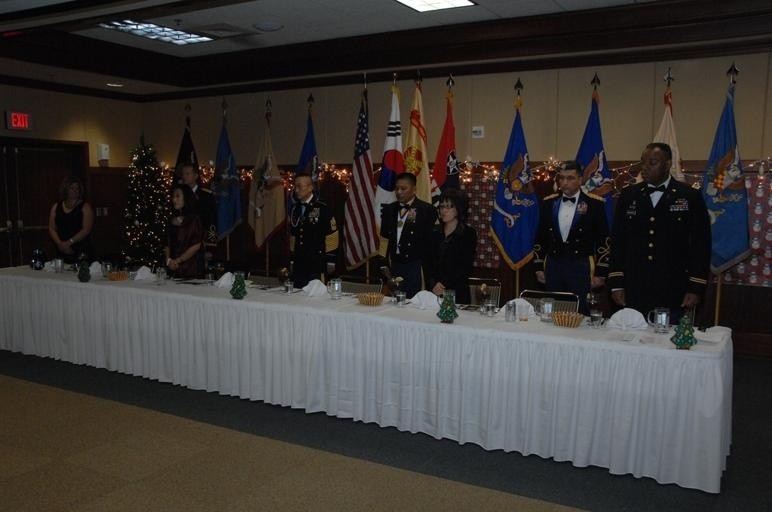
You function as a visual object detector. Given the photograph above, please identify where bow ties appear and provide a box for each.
[398,204,410,211]
[648,185,665,194]
[301,203,308,207]
[562,197,576,204]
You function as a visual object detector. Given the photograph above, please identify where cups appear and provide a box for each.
[284,282,293,295]
[233,271,245,280]
[101,263,110,277]
[535,298,555,321]
[591,311,602,328]
[479,299,496,318]
[395,291,406,306]
[327,278,342,301]
[647,307,670,335]
[55,259,64,272]
[156,267,166,280]
[206,273,214,286]
[437,289,454,307]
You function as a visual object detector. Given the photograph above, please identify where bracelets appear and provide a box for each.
[68,237,76,245]
[166,257,171,267]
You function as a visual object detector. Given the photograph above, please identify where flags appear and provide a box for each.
[488,98,539,267]
[249,97,288,248]
[175,102,199,184]
[403,78,432,205]
[701,85,754,276]
[575,94,616,235]
[374,81,404,257]
[340,83,379,275]
[296,92,318,176]
[433,85,460,189]
[215,91,243,242]
[650,90,685,184]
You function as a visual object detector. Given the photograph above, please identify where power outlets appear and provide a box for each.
[471,126,484,139]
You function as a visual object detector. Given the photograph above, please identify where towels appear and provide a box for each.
[494,298,537,320]
[43,261,70,272]
[134,266,153,280]
[408,290,442,310]
[89,261,104,281]
[605,308,648,331]
[302,279,327,297]
[214,272,236,288]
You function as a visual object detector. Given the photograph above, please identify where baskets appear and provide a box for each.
[106,270,129,281]
[356,292,385,305]
[550,311,584,328]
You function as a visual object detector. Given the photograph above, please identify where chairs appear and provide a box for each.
[340,275,383,294]
[468,278,502,308]
[519,290,579,317]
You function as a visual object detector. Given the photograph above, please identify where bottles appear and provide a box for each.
[31,249,44,269]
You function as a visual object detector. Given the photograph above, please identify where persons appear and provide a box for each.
[287,175,340,278]
[421,190,477,304]
[48,177,95,263]
[164,185,204,278]
[381,173,428,262]
[175,161,217,235]
[533,160,611,317]
[605,142,711,320]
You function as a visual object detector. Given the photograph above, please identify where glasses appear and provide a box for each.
[294,183,312,190]
[439,203,453,209]
